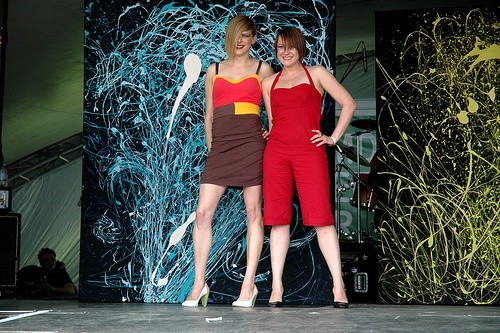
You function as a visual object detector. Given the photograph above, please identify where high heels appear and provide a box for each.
[181,282,210,306]
[269,288,284,307]
[333,293,349,308]
[232,284,259,307]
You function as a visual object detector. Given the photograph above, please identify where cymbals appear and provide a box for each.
[332,142,371,166]
[352,118,377,129]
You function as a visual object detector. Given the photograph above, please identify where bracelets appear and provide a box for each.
[331,136,337,145]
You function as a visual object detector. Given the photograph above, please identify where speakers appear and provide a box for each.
[339,238,380,304]
[0,212,21,288]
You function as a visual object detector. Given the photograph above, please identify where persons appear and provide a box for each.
[182,14,276,307]
[261,26,357,309]
[20,248,77,295]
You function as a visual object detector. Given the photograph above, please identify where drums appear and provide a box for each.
[349,173,377,211]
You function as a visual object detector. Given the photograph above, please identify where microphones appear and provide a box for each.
[362,43,367,72]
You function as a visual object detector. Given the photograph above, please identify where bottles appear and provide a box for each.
[0,160,8,187]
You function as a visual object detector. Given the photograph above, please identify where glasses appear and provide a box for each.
[41,257,55,262]
